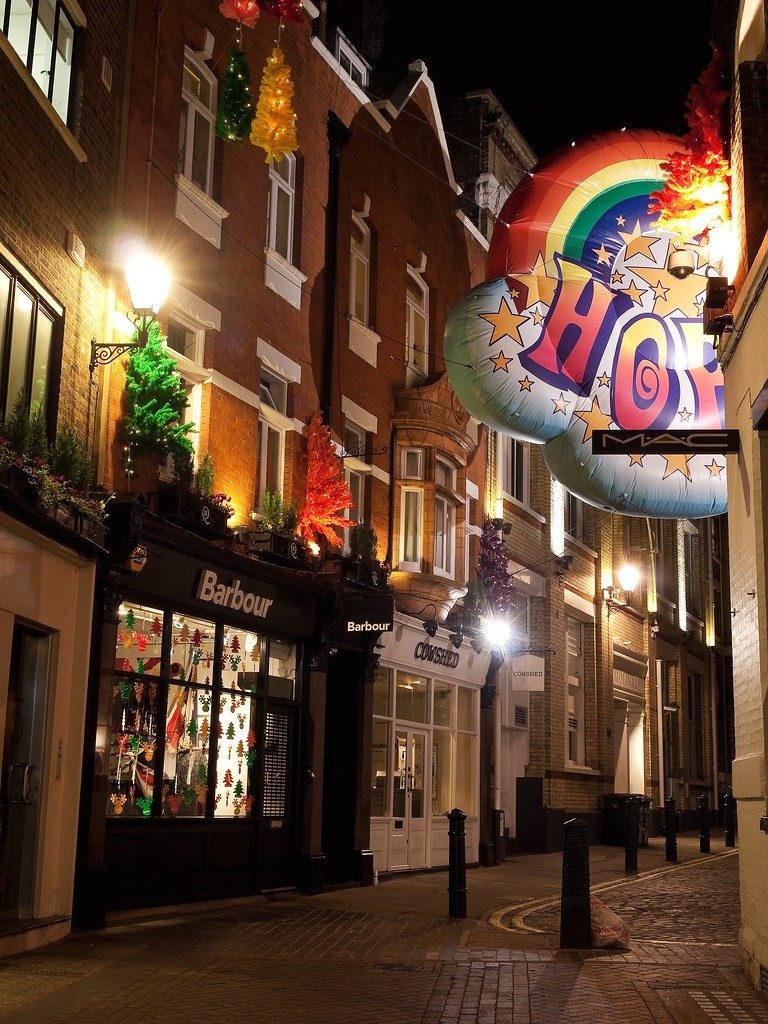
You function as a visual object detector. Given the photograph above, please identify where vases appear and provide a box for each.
[188,499,229,532]
[0,459,109,547]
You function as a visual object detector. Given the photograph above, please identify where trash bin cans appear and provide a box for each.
[601,793,654,847]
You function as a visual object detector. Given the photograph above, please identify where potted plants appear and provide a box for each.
[335,522,390,588]
[257,485,306,567]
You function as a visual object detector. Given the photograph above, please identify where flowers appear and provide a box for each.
[0,434,112,519]
[206,492,233,511]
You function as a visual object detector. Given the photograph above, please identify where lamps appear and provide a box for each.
[89,246,174,380]
[470,639,484,654]
[402,604,439,638]
[439,618,463,649]
